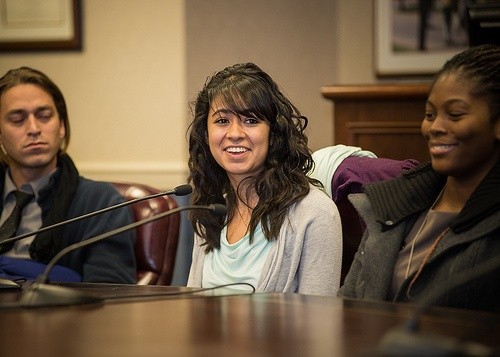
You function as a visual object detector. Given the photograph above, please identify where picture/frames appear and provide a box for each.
[373,0,469,80]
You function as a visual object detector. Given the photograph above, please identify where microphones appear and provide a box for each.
[0,185,193,289]
[18,204,227,308]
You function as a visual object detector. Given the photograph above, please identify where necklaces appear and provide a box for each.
[405,183,450,298]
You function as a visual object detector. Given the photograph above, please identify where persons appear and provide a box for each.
[186,61,343,299]
[337,44,500,314]
[0,66,135,284]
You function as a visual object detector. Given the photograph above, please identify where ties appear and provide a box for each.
[0,191,35,255]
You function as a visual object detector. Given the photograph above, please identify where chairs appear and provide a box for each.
[108,182,181,284]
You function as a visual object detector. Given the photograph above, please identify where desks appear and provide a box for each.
[0,283,500,357]
